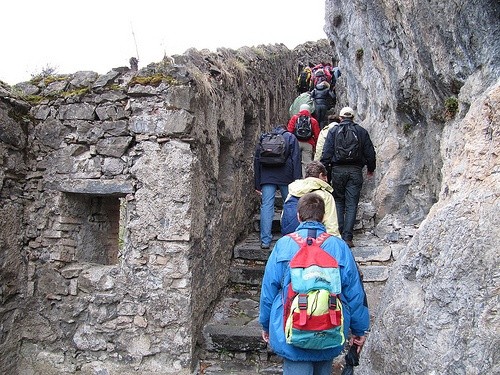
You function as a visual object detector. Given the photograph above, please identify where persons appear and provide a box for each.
[279,161,343,239]
[260,194,369,375]
[288,103,319,179]
[314,115,341,160]
[289,64,341,125]
[320,106,376,248]
[253,125,301,250]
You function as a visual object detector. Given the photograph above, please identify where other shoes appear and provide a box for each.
[261,234,273,249]
[345,240,354,248]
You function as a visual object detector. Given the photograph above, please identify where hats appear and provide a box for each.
[339,107,355,117]
[299,104,311,112]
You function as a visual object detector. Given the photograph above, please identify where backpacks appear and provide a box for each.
[281,195,302,238]
[313,75,326,88]
[334,123,361,163]
[300,71,310,84]
[294,113,313,139]
[259,130,290,166]
[282,232,347,350]
